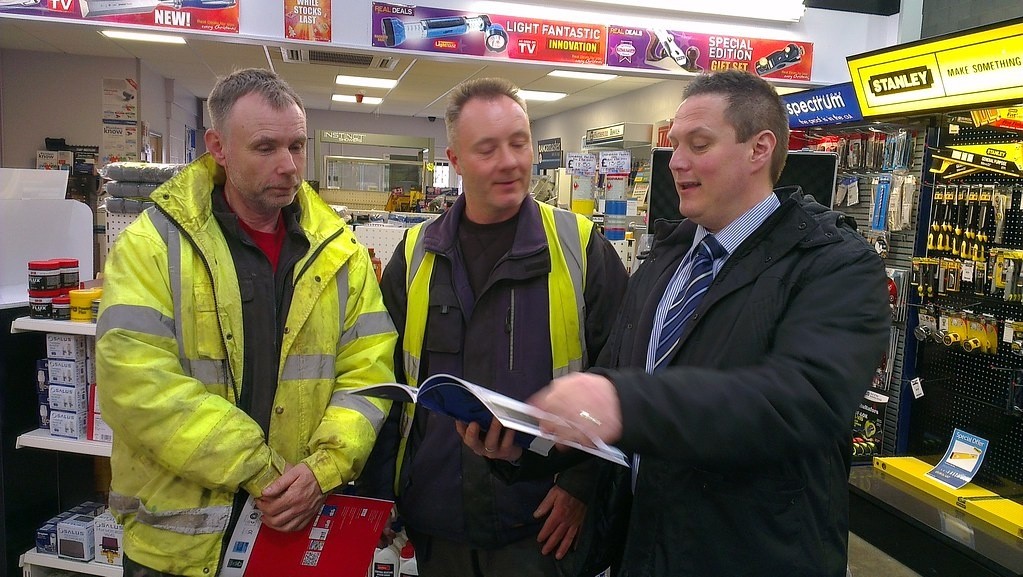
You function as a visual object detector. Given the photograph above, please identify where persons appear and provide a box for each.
[374,80,631,576]
[94,69,398,577]
[455,73,888,577]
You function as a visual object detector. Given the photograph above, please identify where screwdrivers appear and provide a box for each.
[926,200,988,261]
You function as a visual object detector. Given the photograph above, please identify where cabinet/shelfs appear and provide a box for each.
[66,175,100,276]
[9,312,123,577]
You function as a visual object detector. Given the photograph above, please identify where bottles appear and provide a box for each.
[367,248,381,284]
[28,258,80,321]
[68,287,102,323]
[366,518,419,577]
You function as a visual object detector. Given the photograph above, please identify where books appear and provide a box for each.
[348,374,629,468]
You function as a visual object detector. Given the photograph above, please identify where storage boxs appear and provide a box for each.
[35,150,74,174]
[23,334,123,565]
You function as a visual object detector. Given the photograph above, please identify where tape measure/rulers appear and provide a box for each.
[1011,340,1023,356]
[914,325,932,343]
[943,332,960,350]
[934,329,948,343]
[962,337,981,356]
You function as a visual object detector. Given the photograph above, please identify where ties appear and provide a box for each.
[653,235,730,372]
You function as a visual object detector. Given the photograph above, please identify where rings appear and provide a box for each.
[581,412,600,426]
[485,448,496,453]
